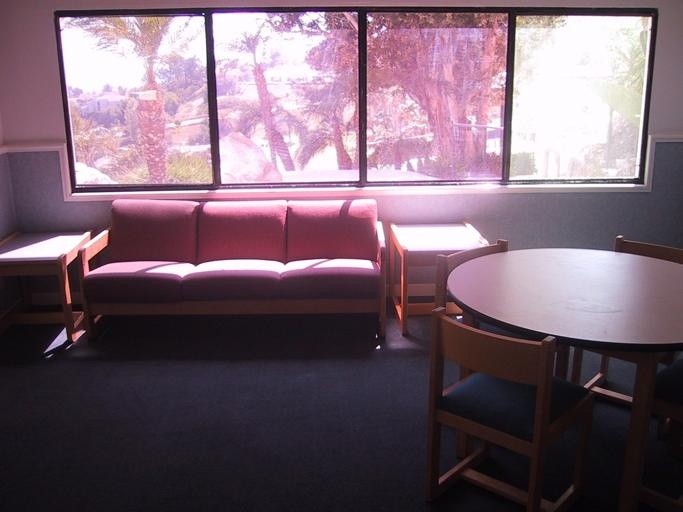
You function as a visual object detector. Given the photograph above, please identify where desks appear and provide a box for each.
[389,219,490,338]
[0,229,93,342]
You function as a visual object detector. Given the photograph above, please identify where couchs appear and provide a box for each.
[79,198,388,340]
